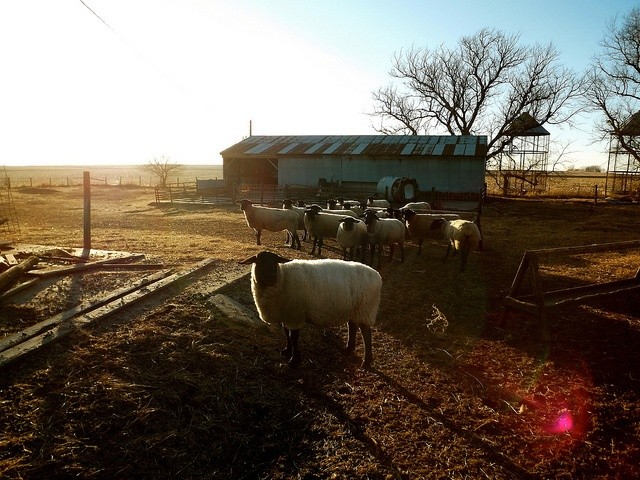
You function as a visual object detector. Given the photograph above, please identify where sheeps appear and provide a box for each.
[237,196,481,273]
[237,251,382,369]
